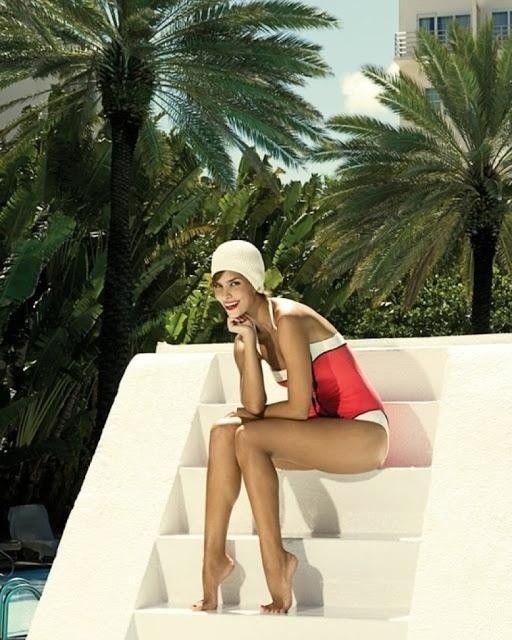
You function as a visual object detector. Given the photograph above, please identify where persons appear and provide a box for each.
[189,238,393,617]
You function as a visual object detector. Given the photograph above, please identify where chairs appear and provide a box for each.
[0,540,21,577]
[9,504,59,565]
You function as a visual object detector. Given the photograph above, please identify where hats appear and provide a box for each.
[211,238,264,294]
